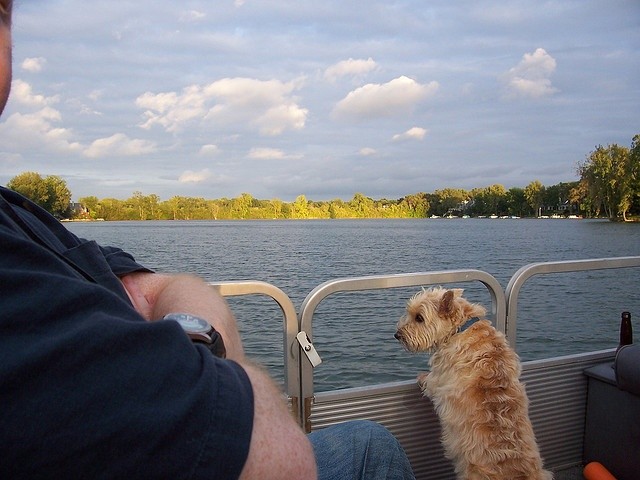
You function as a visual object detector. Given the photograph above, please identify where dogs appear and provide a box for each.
[392,284,552,476]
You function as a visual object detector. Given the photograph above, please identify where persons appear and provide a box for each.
[0,0,418,476]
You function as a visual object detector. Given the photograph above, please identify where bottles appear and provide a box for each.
[615,311,633,357]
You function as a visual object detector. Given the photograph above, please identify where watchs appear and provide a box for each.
[157,311,229,358]
